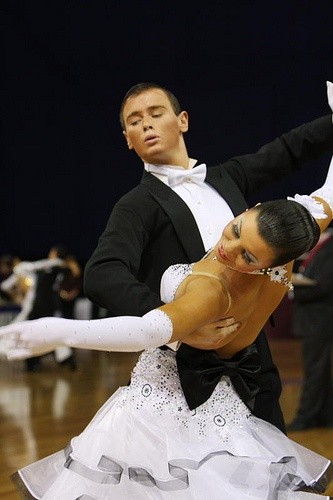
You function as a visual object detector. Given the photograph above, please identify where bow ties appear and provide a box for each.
[144,163,206,188]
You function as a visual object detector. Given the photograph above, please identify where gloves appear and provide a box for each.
[309,81,333,215]
[0,309,173,363]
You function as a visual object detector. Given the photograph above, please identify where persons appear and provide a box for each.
[0,247,81,374]
[285,220,333,432]
[84,84,333,436]
[0,81,333,500]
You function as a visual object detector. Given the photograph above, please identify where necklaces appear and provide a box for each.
[213,253,264,275]
